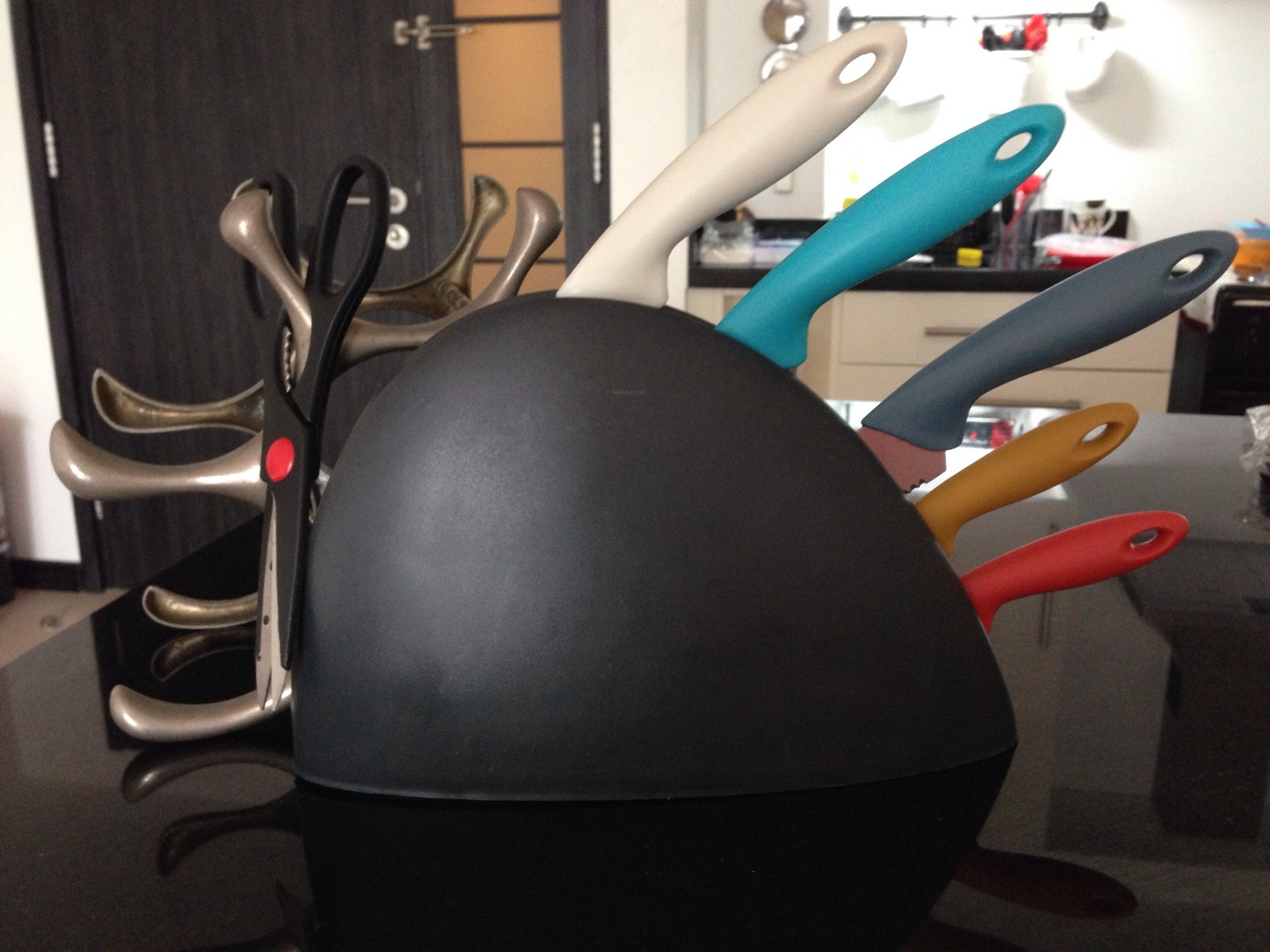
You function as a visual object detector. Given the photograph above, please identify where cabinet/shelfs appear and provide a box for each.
[830,292,1179,413]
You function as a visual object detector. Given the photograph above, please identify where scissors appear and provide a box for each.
[227,154,392,716]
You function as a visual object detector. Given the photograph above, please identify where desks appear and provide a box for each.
[0,401,1270,952]
[1168,267,1270,416]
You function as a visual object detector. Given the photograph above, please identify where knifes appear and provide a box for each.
[555,19,908,310]
[711,104,1067,377]
[958,511,1193,634]
[857,229,1239,494]
[914,403,1140,557]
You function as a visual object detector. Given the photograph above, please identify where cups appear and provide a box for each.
[993,186,1043,270]
[1067,202,1118,238]
